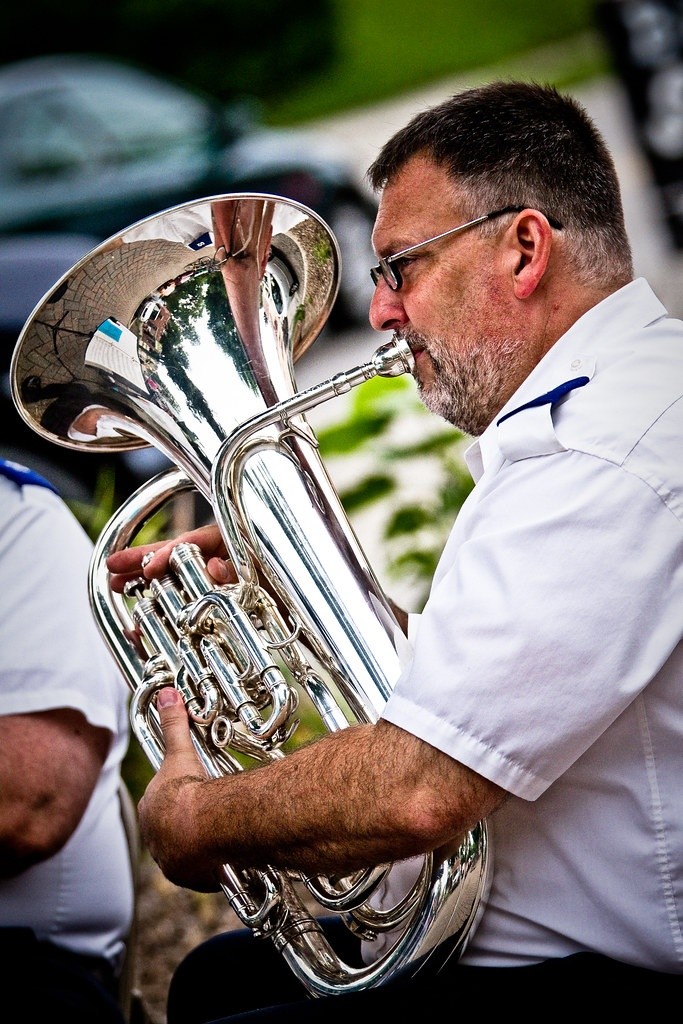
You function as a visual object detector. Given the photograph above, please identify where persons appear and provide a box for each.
[0,456,134,1024]
[103,82,683,1024]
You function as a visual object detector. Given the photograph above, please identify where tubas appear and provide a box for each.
[8,192,493,1002]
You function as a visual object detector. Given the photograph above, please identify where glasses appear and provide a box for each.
[369,203,565,293]
